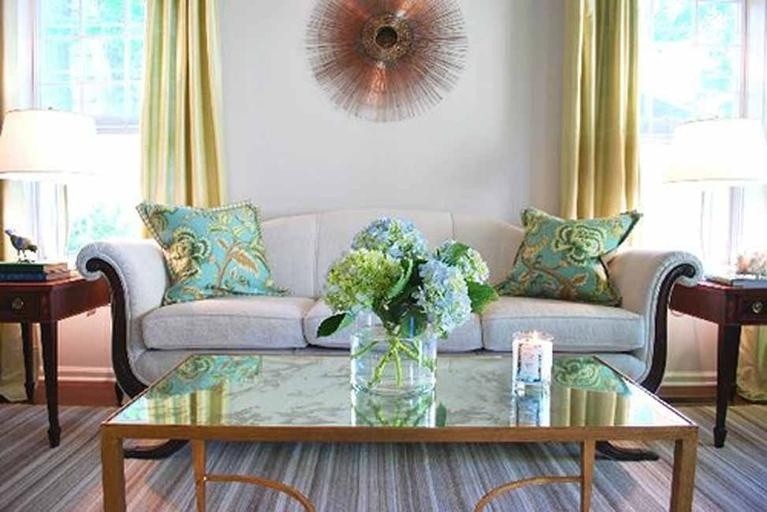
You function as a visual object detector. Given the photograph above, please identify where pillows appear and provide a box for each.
[317,211,453,303]
[454,213,615,296]
[148,353,262,398]
[134,199,290,308]
[484,207,643,308]
[256,215,317,301]
[553,357,631,396]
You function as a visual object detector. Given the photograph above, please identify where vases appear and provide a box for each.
[350,393,436,429]
[349,303,437,395]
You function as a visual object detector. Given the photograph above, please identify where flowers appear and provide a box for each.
[314,216,495,391]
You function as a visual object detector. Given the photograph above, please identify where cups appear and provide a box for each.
[509,386,550,428]
[512,330,555,386]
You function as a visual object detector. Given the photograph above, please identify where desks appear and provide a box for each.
[0,274,112,447]
[669,277,767,448]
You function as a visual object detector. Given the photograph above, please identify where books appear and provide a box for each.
[0,269,73,281]
[703,273,766,286]
[0,262,68,274]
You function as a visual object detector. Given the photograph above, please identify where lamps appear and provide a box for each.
[664,117,767,280]
[0,110,95,279]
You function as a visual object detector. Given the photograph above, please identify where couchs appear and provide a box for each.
[75,212,702,461]
